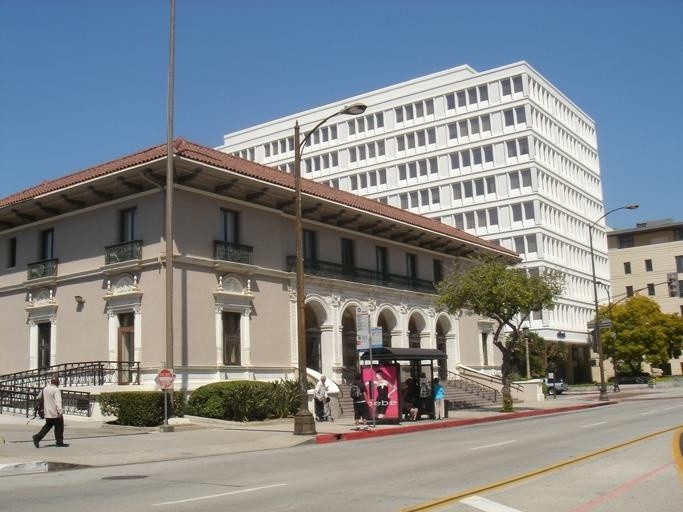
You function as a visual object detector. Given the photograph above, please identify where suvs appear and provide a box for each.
[538,377,567,395]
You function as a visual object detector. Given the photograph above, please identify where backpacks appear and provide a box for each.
[350,384,363,399]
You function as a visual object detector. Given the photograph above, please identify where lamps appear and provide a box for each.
[74,296,85,304]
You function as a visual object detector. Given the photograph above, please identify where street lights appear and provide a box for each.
[588,203,640,401]
[294,102,367,437]
[596,281,620,392]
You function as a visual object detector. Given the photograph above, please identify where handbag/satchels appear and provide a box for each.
[38,397,44,419]
[420,383,430,398]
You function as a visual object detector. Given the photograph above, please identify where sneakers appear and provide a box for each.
[357,427,372,432]
[56,444,69,446]
[32,434,39,448]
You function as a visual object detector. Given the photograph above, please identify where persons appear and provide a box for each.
[313,375,328,422]
[365,370,397,418]
[352,373,370,431]
[31,377,69,448]
[400,372,445,420]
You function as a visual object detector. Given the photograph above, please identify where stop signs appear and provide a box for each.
[154,369,174,390]
